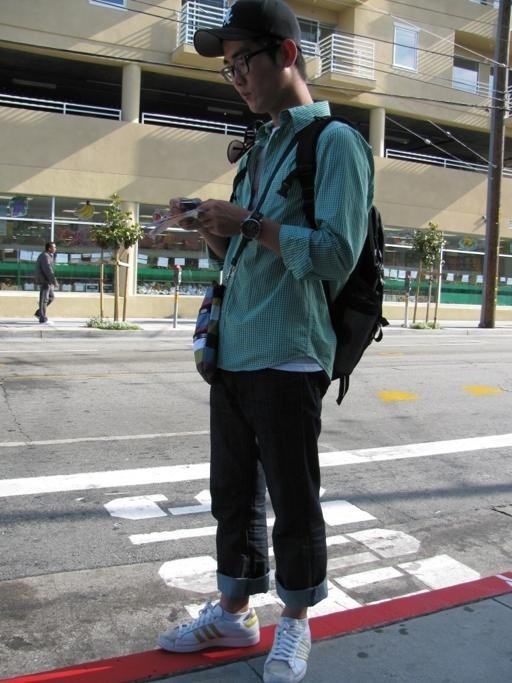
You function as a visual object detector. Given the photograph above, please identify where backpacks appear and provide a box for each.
[296,115,388,380]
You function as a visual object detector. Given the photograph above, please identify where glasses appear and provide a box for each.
[227,121,265,164]
[220,47,270,84]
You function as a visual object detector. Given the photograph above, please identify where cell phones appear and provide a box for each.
[177,198,201,210]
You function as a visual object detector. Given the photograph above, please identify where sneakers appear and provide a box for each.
[35,309,47,322]
[264,616,311,683]
[156,601,259,653]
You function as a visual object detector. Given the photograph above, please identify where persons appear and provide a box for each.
[34,242,59,323]
[154,2,375,683]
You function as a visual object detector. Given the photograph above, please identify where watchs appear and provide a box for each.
[240,211,265,240]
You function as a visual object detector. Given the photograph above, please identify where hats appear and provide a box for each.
[193,1,301,57]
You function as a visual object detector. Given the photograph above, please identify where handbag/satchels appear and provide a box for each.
[192,279,226,385]
